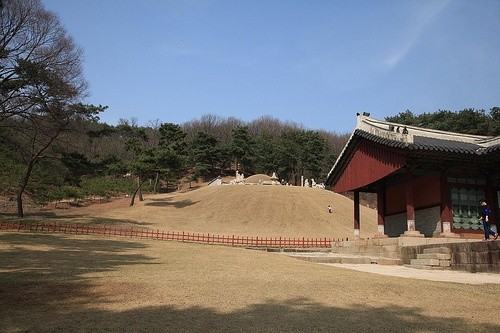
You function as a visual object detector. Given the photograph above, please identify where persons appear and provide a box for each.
[478,199,498,242]
[327,205,332,213]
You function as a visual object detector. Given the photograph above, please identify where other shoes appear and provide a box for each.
[493,233,499,241]
[482,237,491,241]
